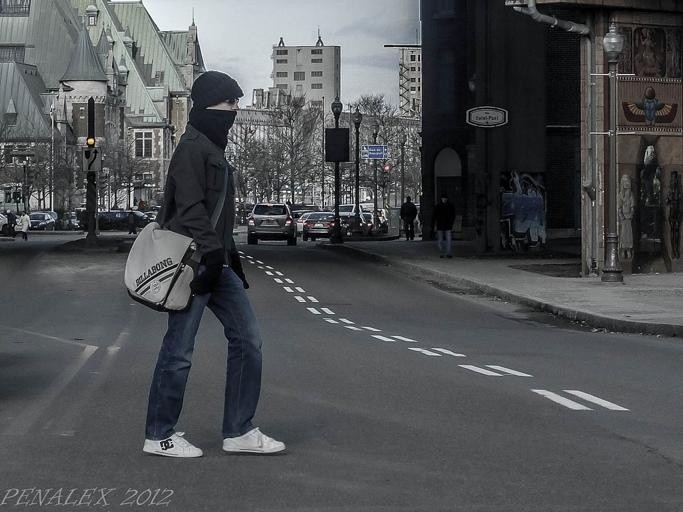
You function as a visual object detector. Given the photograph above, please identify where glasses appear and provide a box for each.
[220,96,240,103]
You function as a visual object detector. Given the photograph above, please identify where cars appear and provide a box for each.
[245,202,389,246]
[0,206,161,235]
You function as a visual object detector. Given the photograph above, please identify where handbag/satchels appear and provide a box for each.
[124,221,199,310]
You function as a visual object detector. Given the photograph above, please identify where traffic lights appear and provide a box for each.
[86,137,94,149]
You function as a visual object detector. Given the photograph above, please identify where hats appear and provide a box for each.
[190,69,244,108]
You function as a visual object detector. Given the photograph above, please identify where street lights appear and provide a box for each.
[603,22,623,281]
[46,97,56,210]
[350,105,364,237]
[371,120,380,235]
[398,128,406,206]
[330,89,346,242]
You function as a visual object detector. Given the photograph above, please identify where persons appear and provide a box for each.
[128,207,137,234]
[142,71,286,458]
[18,211,31,240]
[400,196,417,240]
[6,209,16,238]
[430,195,456,259]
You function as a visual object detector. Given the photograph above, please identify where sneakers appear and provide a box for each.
[141,430,203,459]
[221,428,286,454]
